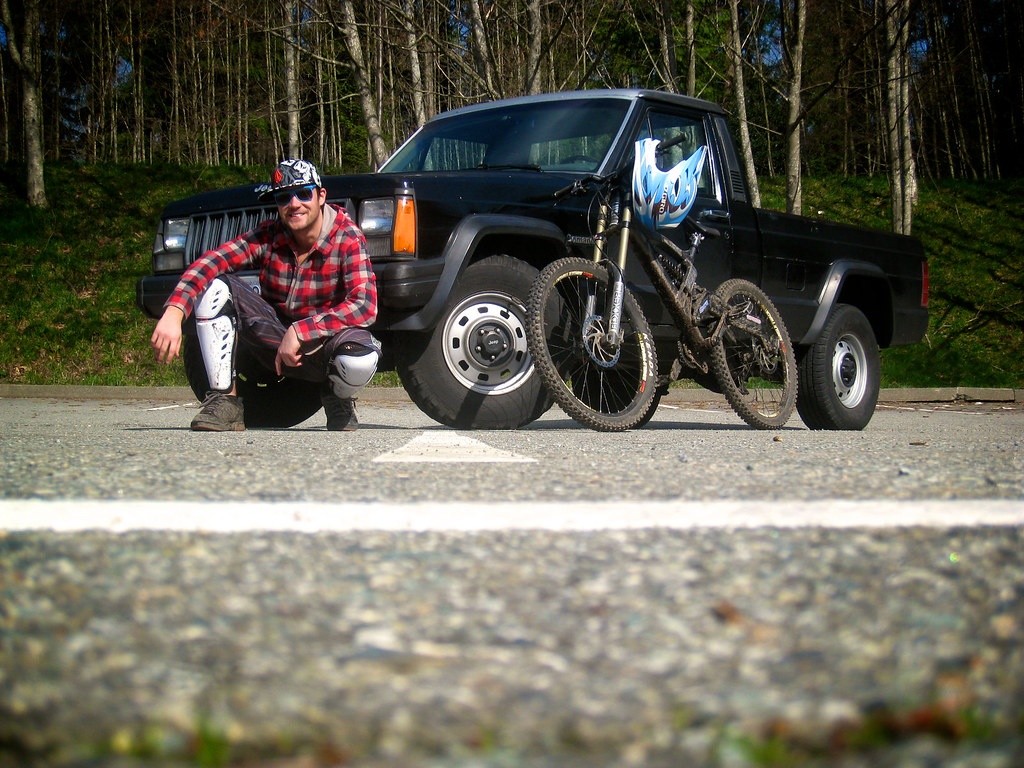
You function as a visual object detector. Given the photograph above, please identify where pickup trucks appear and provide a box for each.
[133,88,929,435]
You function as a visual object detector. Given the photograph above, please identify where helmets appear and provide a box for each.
[630,135,708,230]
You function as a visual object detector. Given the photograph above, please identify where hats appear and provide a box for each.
[255,159,321,201]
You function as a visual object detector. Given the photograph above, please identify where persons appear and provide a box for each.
[150,160,378,435]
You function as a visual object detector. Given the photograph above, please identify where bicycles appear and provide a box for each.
[524,131,800,431]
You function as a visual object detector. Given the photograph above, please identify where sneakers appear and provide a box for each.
[190,392,246,431]
[318,384,359,430]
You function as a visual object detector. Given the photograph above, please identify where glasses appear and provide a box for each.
[273,185,319,207]
